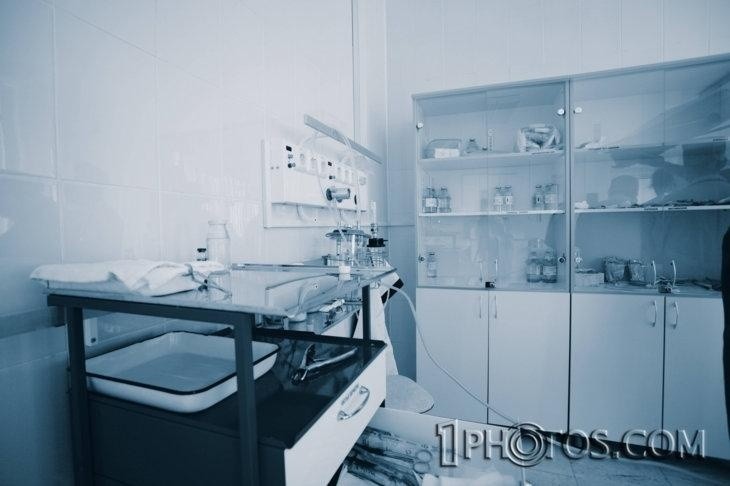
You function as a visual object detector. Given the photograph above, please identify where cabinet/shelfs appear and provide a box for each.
[412,289,570,434]
[45,268,398,486]
[574,52,724,293]
[570,290,730,461]
[412,76,572,291]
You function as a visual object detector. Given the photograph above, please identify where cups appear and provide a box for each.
[604,256,650,282]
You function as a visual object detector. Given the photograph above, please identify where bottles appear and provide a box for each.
[534,184,559,213]
[492,185,514,212]
[197,219,231,268]
[526,250,557,283]
[482,256,498,288]
[467,137,478,154]
[427,252,437,278]
[424,185,449,213]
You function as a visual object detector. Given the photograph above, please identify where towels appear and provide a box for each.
[30,255,226,291]
